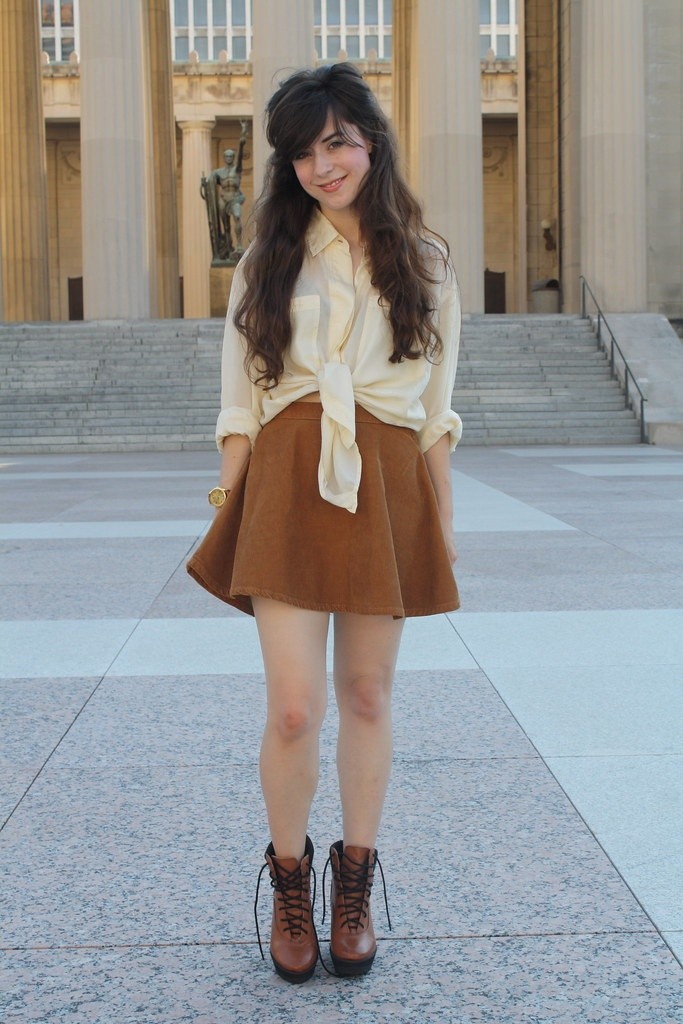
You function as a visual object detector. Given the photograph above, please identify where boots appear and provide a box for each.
[254,834,338,985]
[321,841,391,977]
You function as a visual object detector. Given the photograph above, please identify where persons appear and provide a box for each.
[187,61,463,982]
[201,137,247,259]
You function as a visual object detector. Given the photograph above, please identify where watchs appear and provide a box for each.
[209,487,231,508]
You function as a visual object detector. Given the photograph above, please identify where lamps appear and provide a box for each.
[540,219,557,251]
